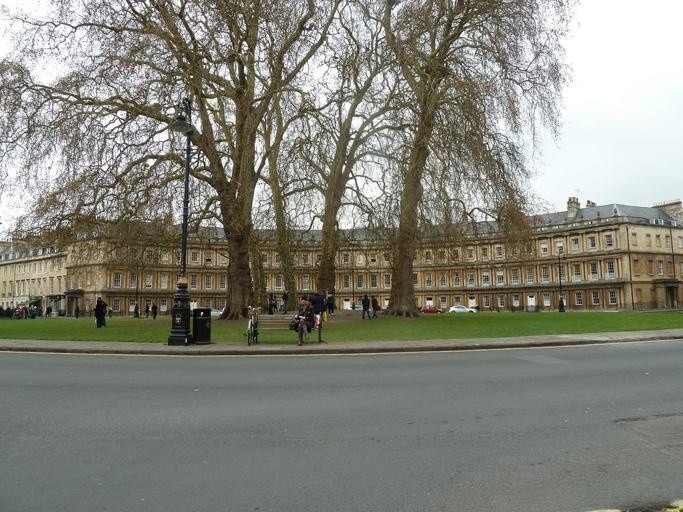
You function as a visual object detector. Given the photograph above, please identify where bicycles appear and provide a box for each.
[241,305,263,345]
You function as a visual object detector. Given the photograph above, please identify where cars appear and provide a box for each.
[420,305,443,314]
[448,305,477,313]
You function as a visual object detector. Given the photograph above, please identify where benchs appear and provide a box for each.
[242,311,322,346]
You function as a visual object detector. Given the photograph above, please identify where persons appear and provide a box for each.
[267,294,278,314]
[134,301,158,320]
[371,295,379,318]
[362,295,371,320]
[93,297,113,328]
[12,305,53,319]
[281,291,289,314]
[292,291,334,347]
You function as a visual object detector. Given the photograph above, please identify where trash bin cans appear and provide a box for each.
[193,309,211,344]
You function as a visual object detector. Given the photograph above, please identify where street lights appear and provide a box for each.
[558,250,566,312]
[167,96,191,347]
